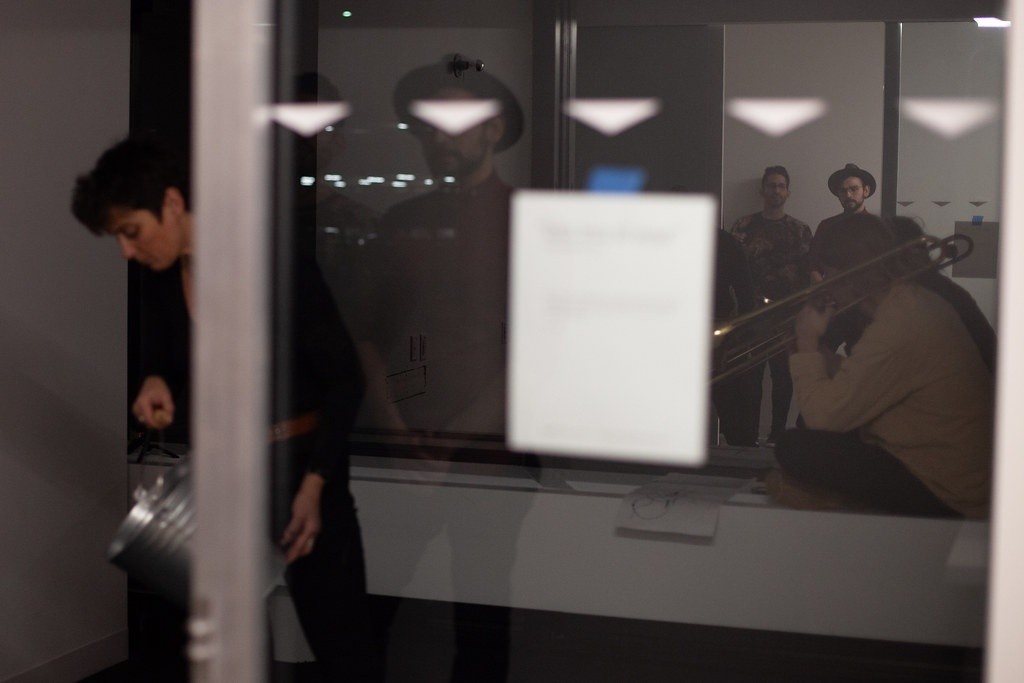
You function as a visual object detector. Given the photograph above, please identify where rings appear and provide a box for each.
[308,538,315,547]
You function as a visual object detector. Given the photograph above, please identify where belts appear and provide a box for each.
[271,414,317,443]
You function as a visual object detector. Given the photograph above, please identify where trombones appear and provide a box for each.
[715,234,974,384]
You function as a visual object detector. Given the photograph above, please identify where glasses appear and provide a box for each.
[836,185,864,197]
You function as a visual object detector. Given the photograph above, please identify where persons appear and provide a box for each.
[774,217,997,517]
[70,132,374,683]
[375,54,525,683]
[808,163,884,354]
[285,70,375,305]
[730,166,814,442]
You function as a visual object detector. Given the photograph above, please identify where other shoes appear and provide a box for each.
[766,470,868,512]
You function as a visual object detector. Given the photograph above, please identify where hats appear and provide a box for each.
[393,54,524,154]
[828,164,876,198]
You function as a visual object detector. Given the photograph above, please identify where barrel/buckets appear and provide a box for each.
[105,411,288,600]
